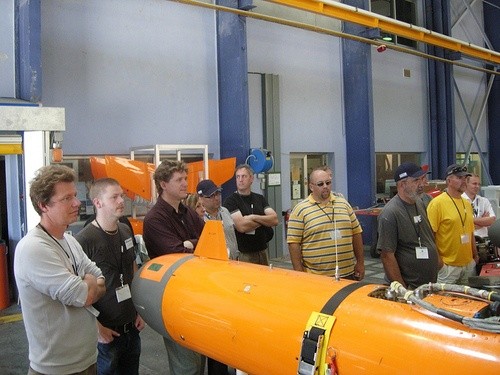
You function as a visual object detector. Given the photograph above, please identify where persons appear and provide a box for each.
[15,166,106,375]
[77,159,496,375]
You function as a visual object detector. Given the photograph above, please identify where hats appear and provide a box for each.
[393,163,432,182]
[196,179,223,197]
[443,164,472,180]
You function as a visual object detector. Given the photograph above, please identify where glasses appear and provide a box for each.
[447,166,467,173]
[204,191,222,198]
[311,181,331,186]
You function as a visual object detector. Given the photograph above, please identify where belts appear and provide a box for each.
[110,319,134,335]
